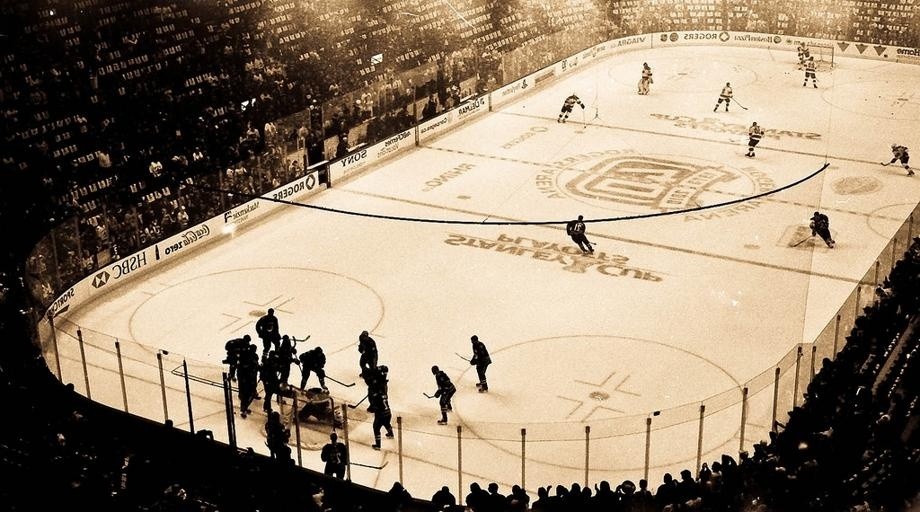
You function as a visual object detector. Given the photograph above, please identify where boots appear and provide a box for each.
[372,433,381,448]
[476,380,488,391]
[438,408,447,422]
[386,427,394,436]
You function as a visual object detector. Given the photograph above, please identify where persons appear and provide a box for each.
[2,0,920,512]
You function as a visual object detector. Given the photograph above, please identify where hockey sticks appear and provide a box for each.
[730,97,750,110]
[280,334,311,342]
[455,352,471,362]
[789,236,813,248]
[423,392,435,398]
[324,375,356,387]
[584,108,587,130]
[348,395,368,409]
[588,241,597,246]
[253,388,266,399]
[347,462,388,469]
[881,162,891,166]
